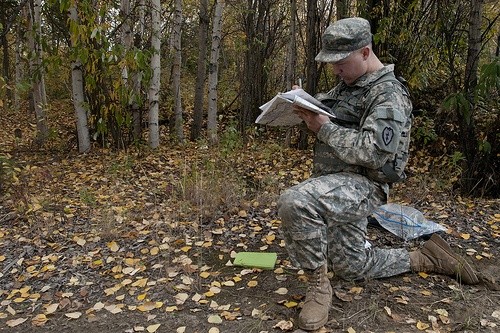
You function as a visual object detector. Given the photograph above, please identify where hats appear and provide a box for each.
[314,16,373,61]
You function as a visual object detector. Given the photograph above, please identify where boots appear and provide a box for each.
[408,233,479,285]
[299,262,333,331]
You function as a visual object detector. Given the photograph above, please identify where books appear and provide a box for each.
[232,251,278,270]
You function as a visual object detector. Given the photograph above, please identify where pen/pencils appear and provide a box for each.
[298,78,302,89]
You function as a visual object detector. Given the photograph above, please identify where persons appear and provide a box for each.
[276,16,481,332]
